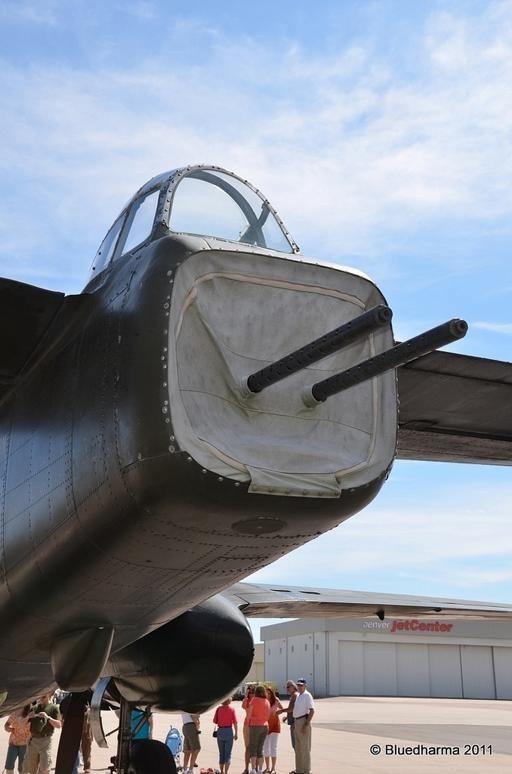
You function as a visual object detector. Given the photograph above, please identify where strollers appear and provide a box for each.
[163,725,184,773]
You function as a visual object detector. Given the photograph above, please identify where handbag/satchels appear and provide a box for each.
[213,731,218,737]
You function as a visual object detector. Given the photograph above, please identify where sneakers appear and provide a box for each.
[242,769,275,774]
[85,769,90,773]
[182,770,194,774]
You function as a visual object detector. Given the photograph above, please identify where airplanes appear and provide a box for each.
[0,158,512,774]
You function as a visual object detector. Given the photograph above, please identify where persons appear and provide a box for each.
[4,676,316,774]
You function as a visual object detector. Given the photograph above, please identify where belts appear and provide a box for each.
[32,733,51,738]
[219,726,231,728]
[296,714,306,719]
[186,722,195,724]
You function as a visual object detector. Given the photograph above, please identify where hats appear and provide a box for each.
[296,678,306,685]
